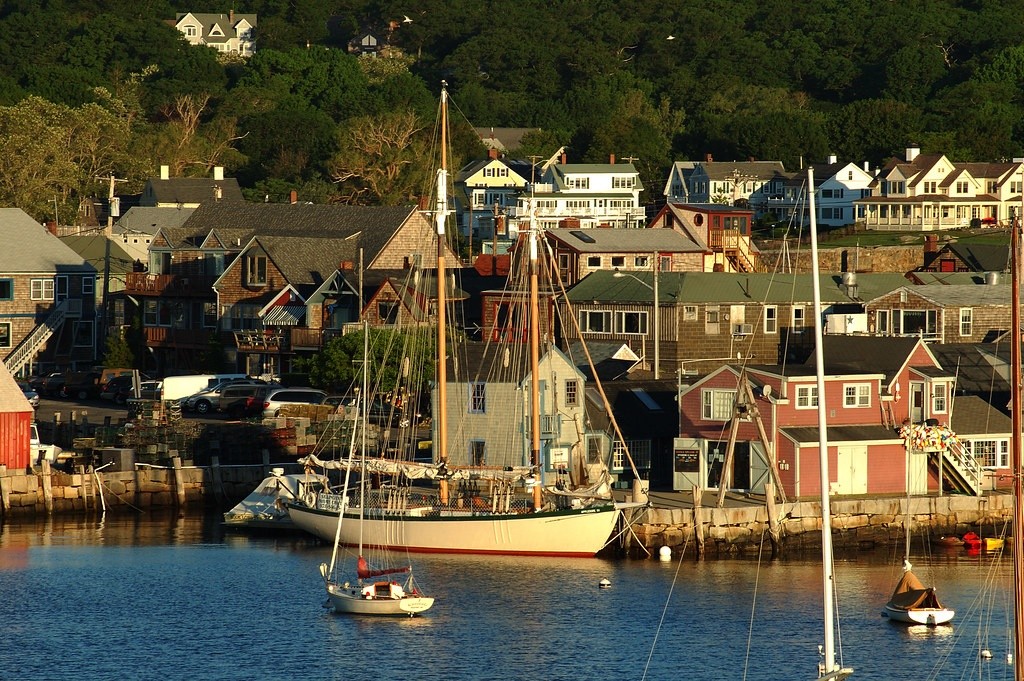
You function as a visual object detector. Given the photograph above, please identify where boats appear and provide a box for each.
[934,534,965,547]
[223,467,343,531]
[963,531,982,546]
[599,577,613,590]
[30,422,62,465]
[984,537,1003,551]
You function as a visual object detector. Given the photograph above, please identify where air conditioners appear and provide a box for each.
[740,324,752,333]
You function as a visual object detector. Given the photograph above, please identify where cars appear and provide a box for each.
[15,381,41,410]
[26,365,339,420]
[320,395,423,429]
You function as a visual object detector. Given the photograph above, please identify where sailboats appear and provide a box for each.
[881,386,956,626]
[319,318,436,615]
[275,75,655,559]
